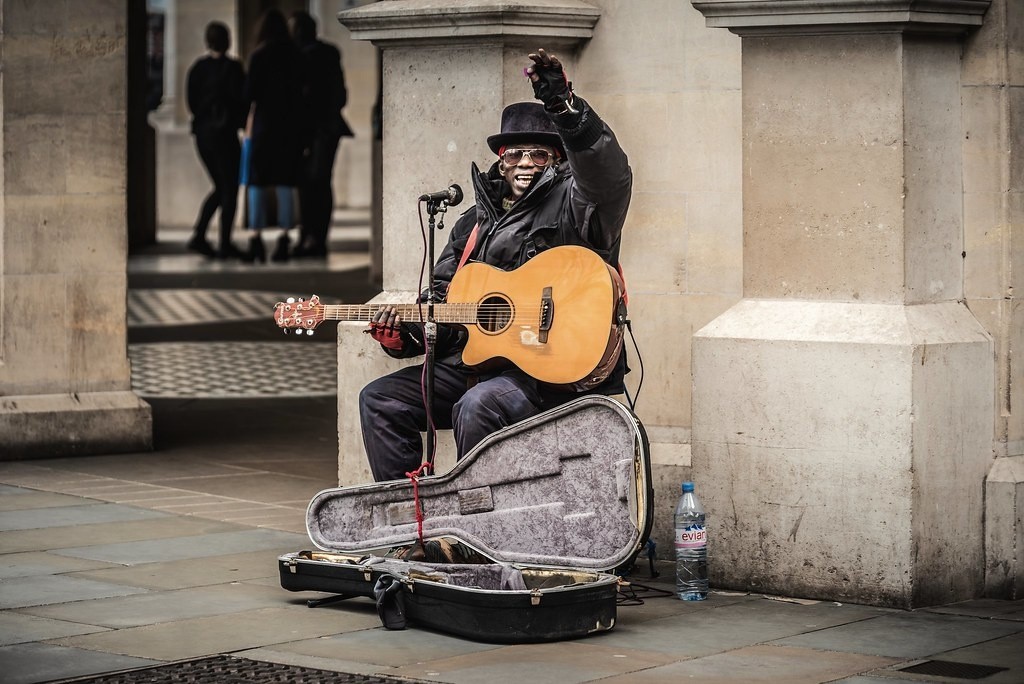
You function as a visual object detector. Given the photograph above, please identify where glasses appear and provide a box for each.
[501,149,554,167]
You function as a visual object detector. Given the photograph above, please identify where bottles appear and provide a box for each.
[674,481,708,600]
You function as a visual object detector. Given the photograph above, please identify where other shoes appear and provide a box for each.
[217,242,244,260]
[187,237,218,258]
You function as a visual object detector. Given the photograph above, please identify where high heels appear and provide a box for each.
[241,236,266,263]
[272,236,291,262]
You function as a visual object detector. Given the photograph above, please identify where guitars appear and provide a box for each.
[271,244,624,392]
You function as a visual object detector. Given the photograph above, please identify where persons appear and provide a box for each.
[357,45,632,561]
[184,10,357,264]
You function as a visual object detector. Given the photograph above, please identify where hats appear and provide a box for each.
[487,102,568,164]
